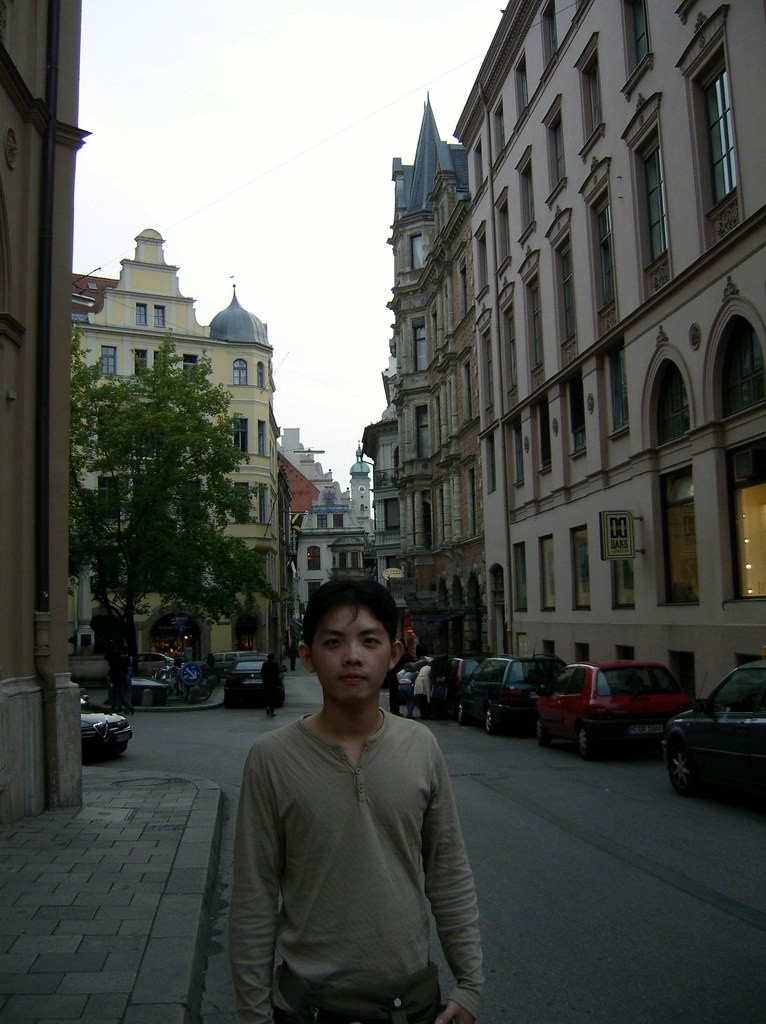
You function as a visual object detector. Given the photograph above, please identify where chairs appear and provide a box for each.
[502,670,516,679]
[627,674,644,686]
[527,669,546,680]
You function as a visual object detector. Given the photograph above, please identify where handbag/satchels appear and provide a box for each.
[396,669,406,682]
[430,681,448,701]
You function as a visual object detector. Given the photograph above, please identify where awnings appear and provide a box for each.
[425,613,464,622]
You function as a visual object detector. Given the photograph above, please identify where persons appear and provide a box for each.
[289,643,299,671]
[108,645,137,715]
[389,653,465,720]
[170,641,185,663]
[228,571,484,1024]
[262,653,282,717]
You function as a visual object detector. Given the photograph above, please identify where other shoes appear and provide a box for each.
[130,707,134,715]
[396,713,404,717]
[271,714,275,717]
[266,709,270,715]
[406,715,418,720]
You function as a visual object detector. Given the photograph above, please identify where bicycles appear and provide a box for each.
[150,662,215,702]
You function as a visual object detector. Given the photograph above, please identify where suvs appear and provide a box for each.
[212,650,256,673]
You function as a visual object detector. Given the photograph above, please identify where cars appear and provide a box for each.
[661,659,766,800]
[80,693,134,755]
[429,654,490,717]
[221,656,288,707]
[138,652,175,674]
[533,660,691,762]
[455,652,578,736]
